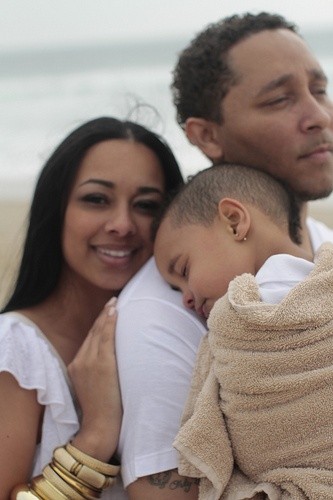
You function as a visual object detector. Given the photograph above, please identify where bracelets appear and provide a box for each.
[8,438,123,500]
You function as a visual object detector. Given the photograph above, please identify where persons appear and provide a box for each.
[150,159,331,500]
[2,116,188,500]
[115,9,333,500]
